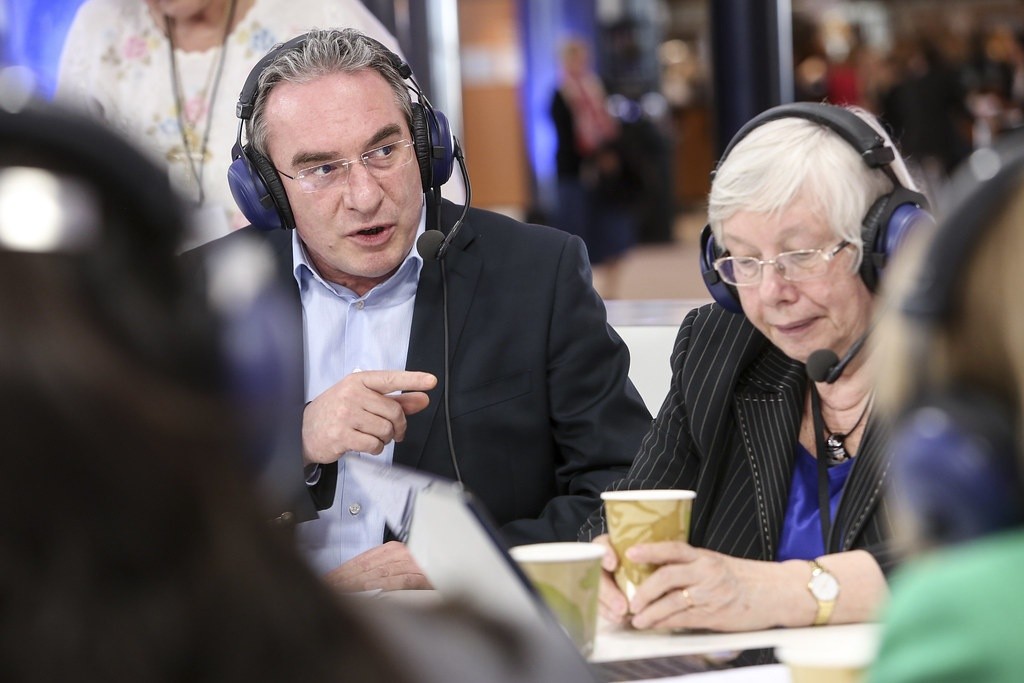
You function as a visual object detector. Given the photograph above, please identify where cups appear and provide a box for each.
[507,541,609,660]
[601,489,698,621]
[774,631,879,683]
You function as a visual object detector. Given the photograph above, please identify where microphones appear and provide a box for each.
[415,158,472,261]
[804,328,872,385]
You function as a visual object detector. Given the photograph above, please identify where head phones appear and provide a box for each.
[227,30,464,231]
[698,101,940,314]
[1,99,284,480]
[885,152,1023,550]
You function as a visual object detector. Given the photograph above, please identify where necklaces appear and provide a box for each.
[162,2,234,215]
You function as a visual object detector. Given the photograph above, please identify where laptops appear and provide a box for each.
[344,456,785,683]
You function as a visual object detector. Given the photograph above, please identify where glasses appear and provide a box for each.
[276,132,415,193]
[711,237,850,286]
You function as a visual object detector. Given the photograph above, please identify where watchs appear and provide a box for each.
[805,559,841,625]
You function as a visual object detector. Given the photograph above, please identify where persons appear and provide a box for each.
[190,31,652,594]
[0,103,559,683]
[861,146,1024,683]
[548,0,1024,247]
[577,101,948,633]
[58,2,423,259]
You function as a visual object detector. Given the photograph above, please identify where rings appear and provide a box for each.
[682,588,694,609]
